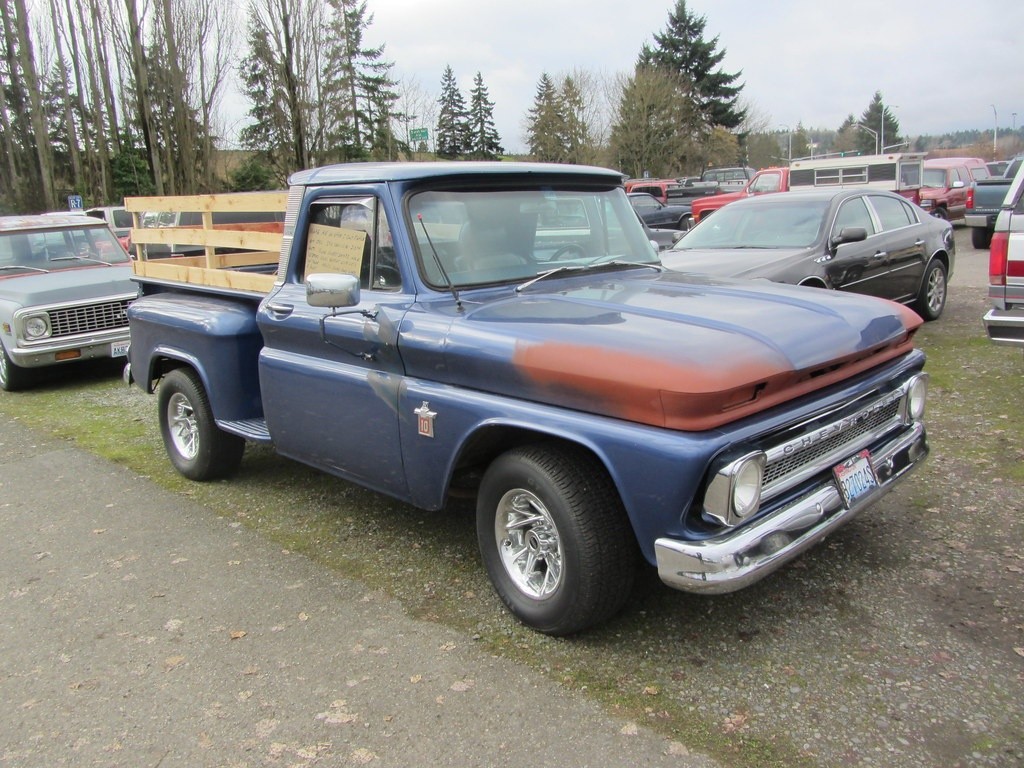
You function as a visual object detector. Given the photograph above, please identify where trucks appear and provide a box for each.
[689,153,931,249]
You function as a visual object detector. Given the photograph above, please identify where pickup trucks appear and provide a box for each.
[118,160,933,644]
[963,156,1024,247]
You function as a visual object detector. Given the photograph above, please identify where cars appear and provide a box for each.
[986,160,1012,179]
[982,161,1024,350]
[657,184,953,323]
[623,165,759,233]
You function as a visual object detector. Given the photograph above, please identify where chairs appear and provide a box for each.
[454,218,527,272]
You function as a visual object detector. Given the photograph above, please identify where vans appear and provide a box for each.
[0,211,149,393]
[914,158,991,222]
[86,206,145,259]
[127,207,288,266]
